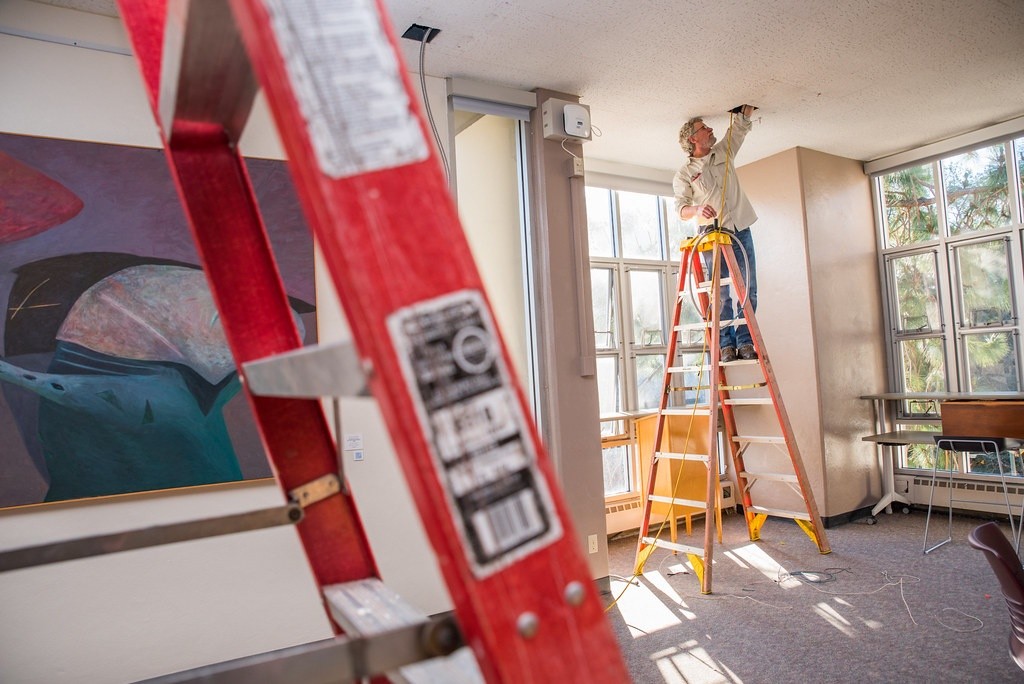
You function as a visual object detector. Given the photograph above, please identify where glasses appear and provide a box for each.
[690,123,708,138]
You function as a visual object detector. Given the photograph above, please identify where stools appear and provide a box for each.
[924,436,1024,557]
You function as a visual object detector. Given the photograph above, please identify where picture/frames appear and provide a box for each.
[0,130,341,517]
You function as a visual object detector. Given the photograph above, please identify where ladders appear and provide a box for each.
[120,1,638,683]
[631,233,834,594]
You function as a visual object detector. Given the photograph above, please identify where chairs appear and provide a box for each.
[968,521,1024,673]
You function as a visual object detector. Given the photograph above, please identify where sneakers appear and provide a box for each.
[738,345,760,360]
[720,346,737,363]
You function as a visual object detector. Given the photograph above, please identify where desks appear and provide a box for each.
[861,392,1024,525]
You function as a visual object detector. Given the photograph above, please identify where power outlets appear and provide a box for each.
[588,534,598,554]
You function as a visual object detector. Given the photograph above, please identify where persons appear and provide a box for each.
[673,104,757,363]
